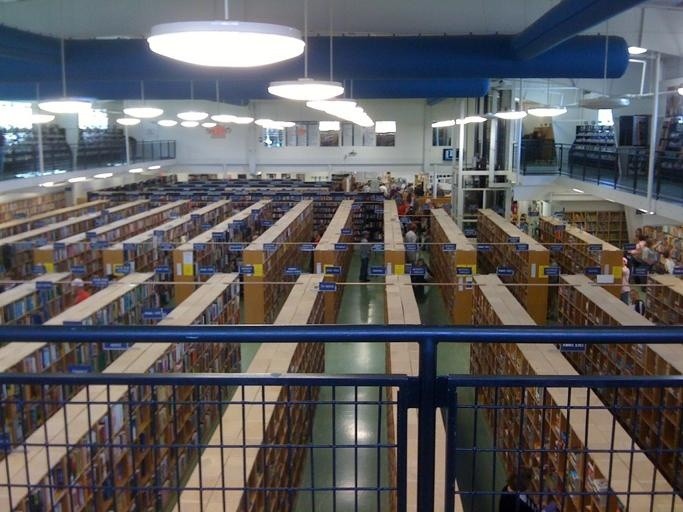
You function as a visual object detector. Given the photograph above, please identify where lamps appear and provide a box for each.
[429,78,568,128]
[0,77,301,138]
[36,31,93,115]
[266,1,344,101]
[305,81,374,128]
[146,0,306,69]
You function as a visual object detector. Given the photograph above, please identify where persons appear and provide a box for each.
[232,250,243,301]
[618,240,677,315]
[308,229,323,274]
[498,463,552,511]
[355,169,435,282]
[69,275,91,306]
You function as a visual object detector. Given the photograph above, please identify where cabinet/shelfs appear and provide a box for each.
[0,182,681,510]
[569,109,682,187]
